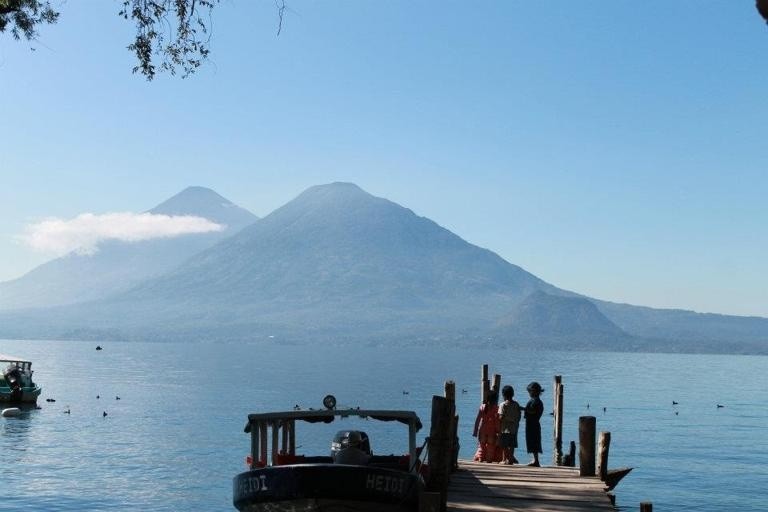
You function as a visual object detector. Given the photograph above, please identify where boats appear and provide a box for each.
[233,409,421,511]
[0,360,41,403]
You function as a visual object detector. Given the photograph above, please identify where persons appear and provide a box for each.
[519,382,545,467]
[472,390,506,464]
[496,386,521,465]
